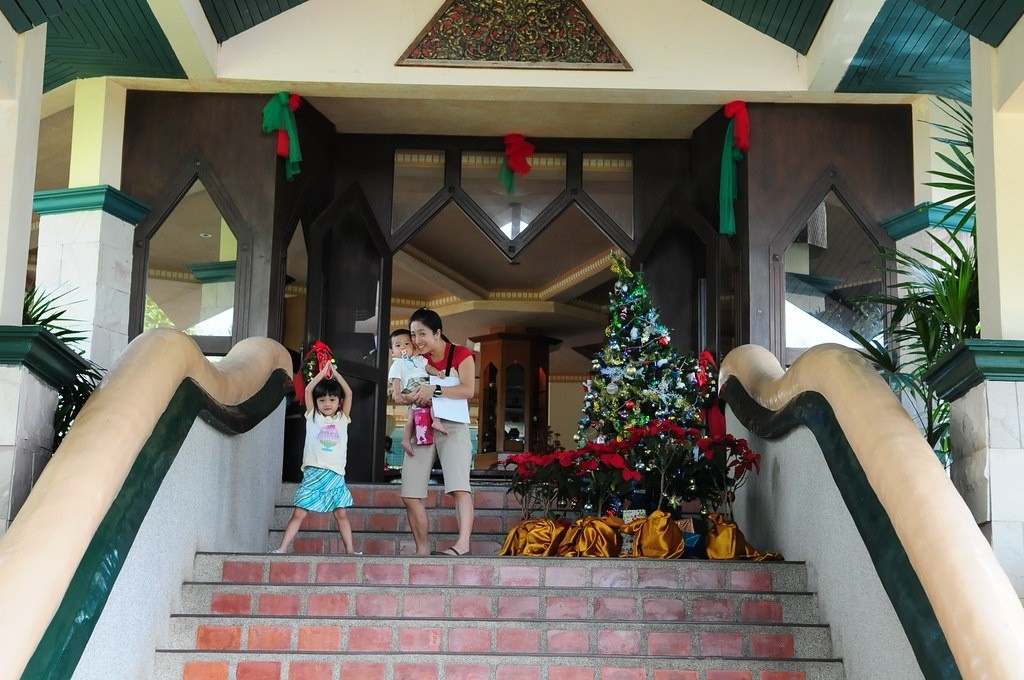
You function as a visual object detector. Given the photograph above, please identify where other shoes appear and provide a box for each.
[431,547,471,556]
[267,549,287,553]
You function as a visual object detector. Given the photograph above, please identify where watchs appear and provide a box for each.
[432,384,442,398]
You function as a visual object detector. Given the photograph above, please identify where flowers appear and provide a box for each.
[486,415,760,520]
[301,350,337,385]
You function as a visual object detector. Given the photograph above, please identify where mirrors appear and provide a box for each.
[503,363,525,453]
[482,362,498,453]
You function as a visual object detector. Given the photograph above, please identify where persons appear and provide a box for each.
[391,307,478,557]
[386,325,452,458]
[268,359,367,554]
